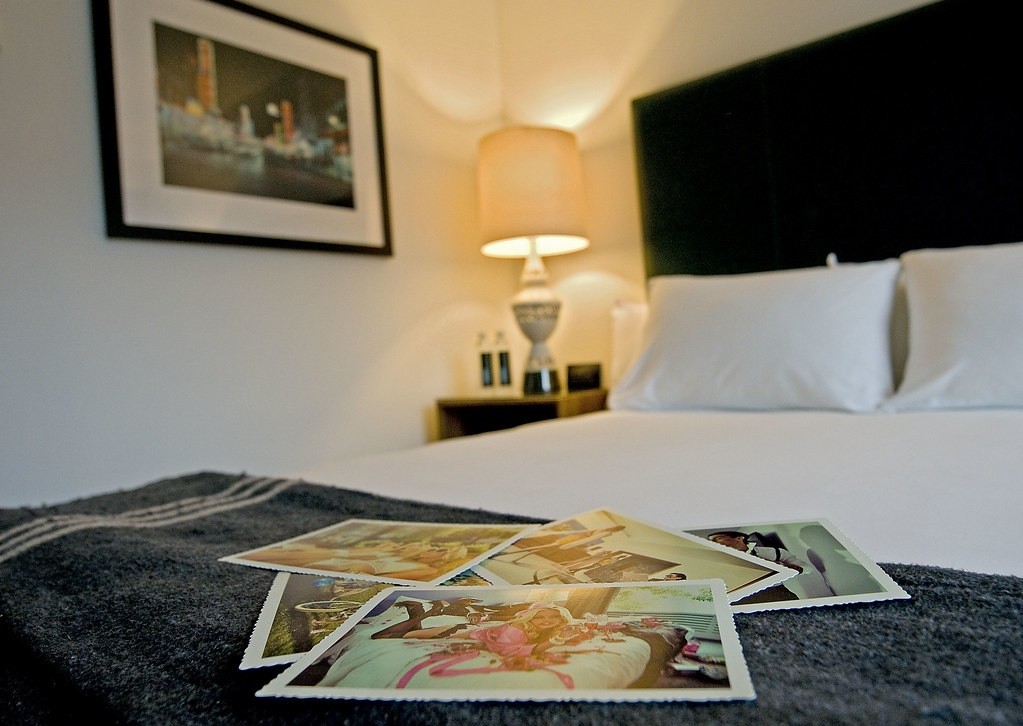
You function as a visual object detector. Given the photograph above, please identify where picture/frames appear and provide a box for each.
[91,0,394,260]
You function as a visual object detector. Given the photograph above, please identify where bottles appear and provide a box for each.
[477,331,512,386]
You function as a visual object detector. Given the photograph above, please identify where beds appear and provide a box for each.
[0,0,1023,726]
[312,596,695,710]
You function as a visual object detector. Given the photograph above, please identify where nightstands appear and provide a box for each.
[435,388,606,442]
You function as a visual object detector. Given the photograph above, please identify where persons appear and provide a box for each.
[648,573,687,582]
[709,531,833,605]
[371,599,579,656]
[488,525,626,570]
[238,543,467,579]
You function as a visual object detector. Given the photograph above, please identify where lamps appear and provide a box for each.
[477,125,590,395]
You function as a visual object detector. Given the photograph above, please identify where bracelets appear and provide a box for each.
[549,636,570,645]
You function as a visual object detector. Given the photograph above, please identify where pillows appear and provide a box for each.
[879,242,1023,412]
[605,259,900,413]
